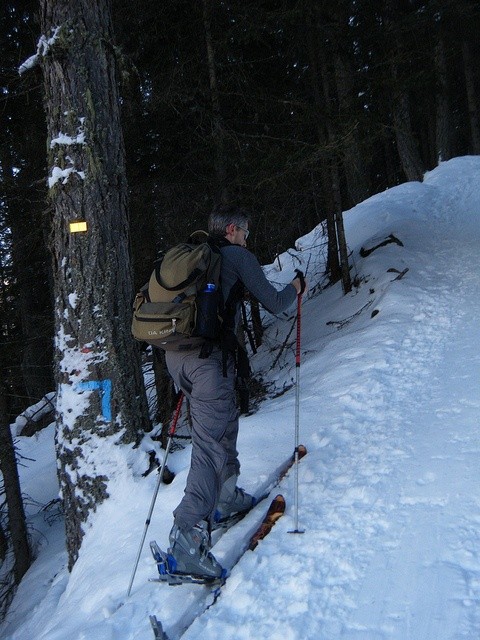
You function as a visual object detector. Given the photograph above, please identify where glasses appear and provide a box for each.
[236,225,249,239]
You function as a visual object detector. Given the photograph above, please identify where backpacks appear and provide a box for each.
[130,230,255,414]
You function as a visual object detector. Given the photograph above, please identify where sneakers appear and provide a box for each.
[214,477,254,523]
[168,519,221,580]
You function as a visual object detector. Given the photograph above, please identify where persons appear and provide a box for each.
[164,207,306,580]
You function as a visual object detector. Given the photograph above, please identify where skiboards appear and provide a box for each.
[150,436,308,640]
